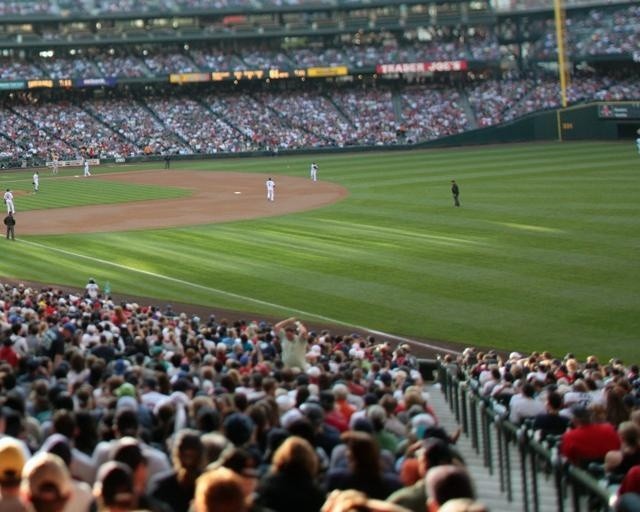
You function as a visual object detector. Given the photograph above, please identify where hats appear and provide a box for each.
[460,346,639,419]
[0,321,450,503]
